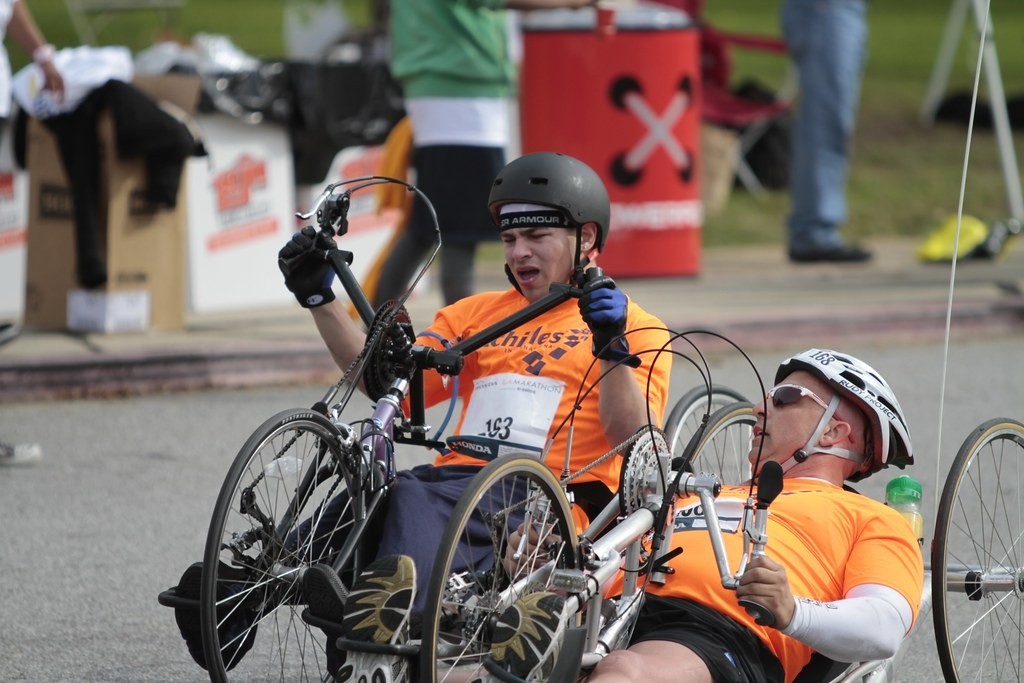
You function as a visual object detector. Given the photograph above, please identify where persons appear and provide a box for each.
[366,0,518,310]
[178,151,923,683]
[781,0,873,263]
[0,1,65,346]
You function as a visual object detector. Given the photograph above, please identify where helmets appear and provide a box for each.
[486,151,611,253]
[773,348,914,483]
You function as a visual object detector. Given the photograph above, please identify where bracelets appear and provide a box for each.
[32,45,56,63]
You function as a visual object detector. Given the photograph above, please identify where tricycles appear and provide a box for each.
[417,326,1024,683]
[155,174,757,683]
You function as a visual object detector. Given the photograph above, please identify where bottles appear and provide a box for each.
[885,473,924,550]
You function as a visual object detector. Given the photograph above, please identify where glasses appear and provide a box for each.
[766,383,855,444]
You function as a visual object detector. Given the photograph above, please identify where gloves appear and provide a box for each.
[576,269,643,369]
[278,226,339,308]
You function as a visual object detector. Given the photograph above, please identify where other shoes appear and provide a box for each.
[1,440,43,466]
[175,560,257,672]
[789,242,872,264]
[305,563,348,620]
[336,554,418,683]
[471,590,569,683]
